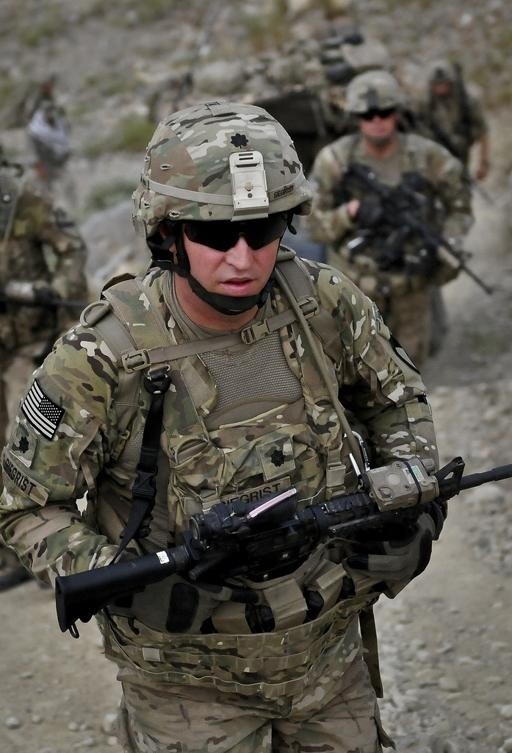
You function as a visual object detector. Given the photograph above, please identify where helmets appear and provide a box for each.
[132,103,311,232]
[343,70,408,115]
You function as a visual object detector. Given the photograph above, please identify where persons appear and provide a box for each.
[306,37,487,219]
[300,69,470,373]
[0,96,443,753]
[1,143,90,590]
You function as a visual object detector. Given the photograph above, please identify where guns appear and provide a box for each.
[341,160,494,297]
[0,278,92,335]
[55,458,512,639]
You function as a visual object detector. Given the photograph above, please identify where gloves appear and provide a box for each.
[353,194,403,238]
[343,512,440,582]
[127,574,259,637]
[407,244,449,288]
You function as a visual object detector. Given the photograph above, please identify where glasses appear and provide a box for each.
[185,211,293,251]
[356,108,398,121]
